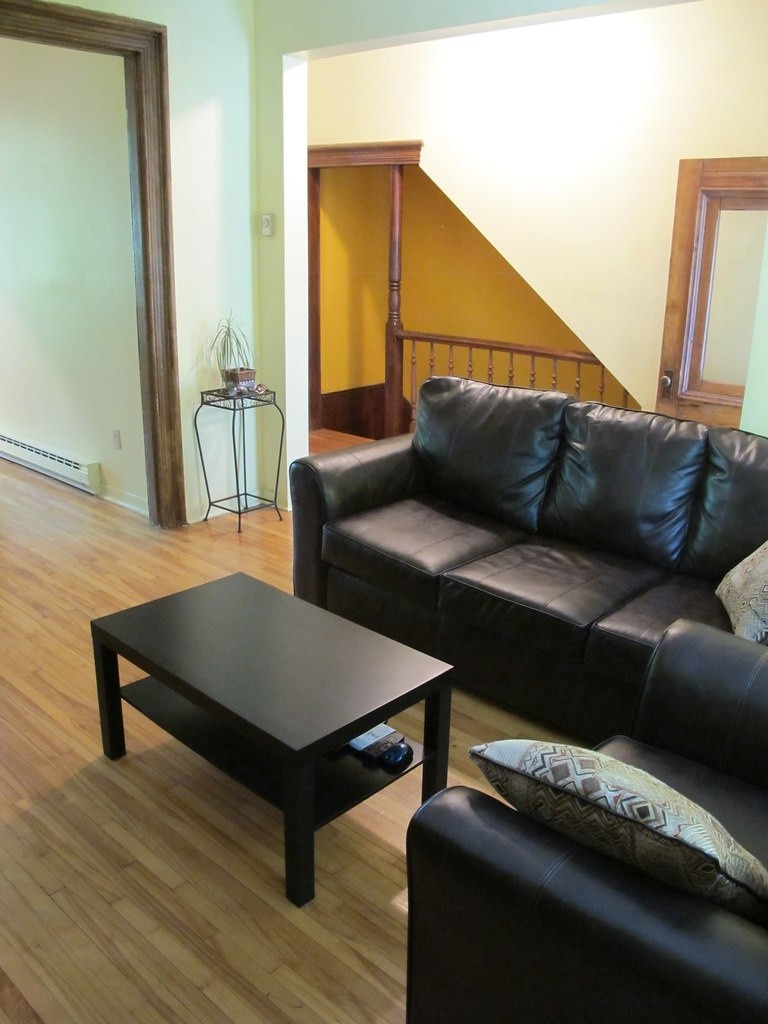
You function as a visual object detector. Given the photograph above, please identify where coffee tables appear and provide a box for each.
[91,572,458,909]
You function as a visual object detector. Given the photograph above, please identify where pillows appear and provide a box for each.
[471,735,768,912]
[715,539,768,642]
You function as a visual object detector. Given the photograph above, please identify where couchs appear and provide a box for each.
[281,375,768,735]
[404,621,768,1024]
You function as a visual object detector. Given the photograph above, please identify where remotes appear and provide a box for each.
[349,722,395,751]
[359,732,406,762]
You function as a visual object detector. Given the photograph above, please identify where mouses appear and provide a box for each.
[385,742,413,771]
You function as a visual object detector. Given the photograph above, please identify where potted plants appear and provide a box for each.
[210,311,257,390]
